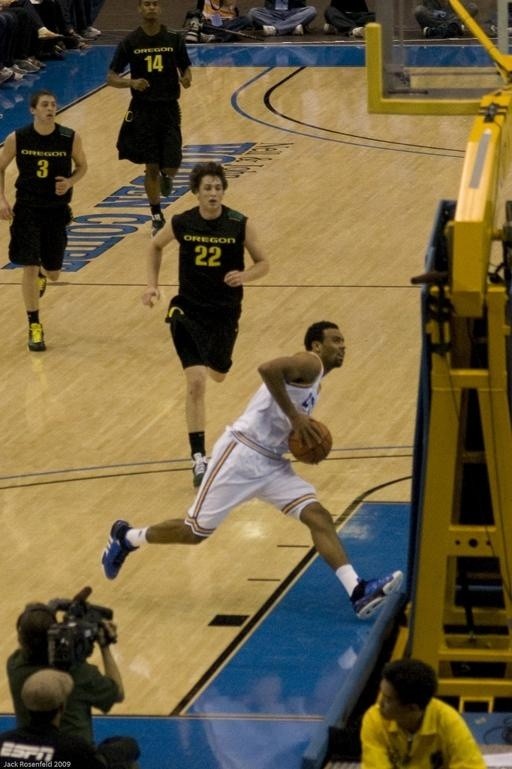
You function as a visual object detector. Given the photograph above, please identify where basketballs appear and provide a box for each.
[288,420,331,464]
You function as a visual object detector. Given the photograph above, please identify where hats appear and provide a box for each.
[21,669,74,710]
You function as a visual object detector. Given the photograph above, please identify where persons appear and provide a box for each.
[107,0,192,237]
[0,667,107,768]
[5,601,125,745]
[0,90,87,353]
[0,0,512,86]
[100,319,404,621]
[97,735,141,769]
[359,658,490,769]
[141,161,270,489]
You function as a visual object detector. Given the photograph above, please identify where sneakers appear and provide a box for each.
[352,570,403,621]
[192,453,208,486]
[160,173,172,197]
[29,323,46,351]
[0,26,101,83]
[151,214,165,238]
[102,520,135,580]
[38,273,46,298]
